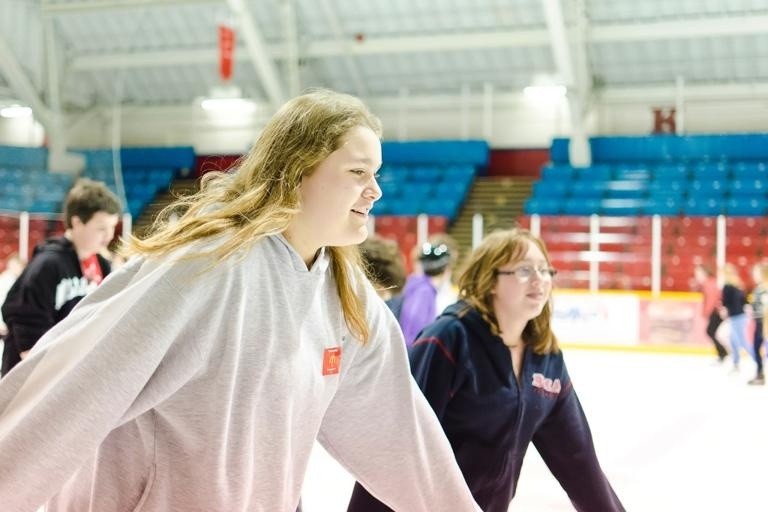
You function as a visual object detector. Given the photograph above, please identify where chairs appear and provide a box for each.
[2,133,767,296]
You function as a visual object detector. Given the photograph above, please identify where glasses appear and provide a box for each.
[495,266,557,283]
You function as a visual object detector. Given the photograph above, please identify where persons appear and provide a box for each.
[356,232,407,302]
[0,176,120,377]
[399,230,457,346]
[691,256,768,384]
[347,220,626,512]
[0,89,483,512]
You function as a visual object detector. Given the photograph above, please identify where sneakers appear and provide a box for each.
[716,355,765,385]
